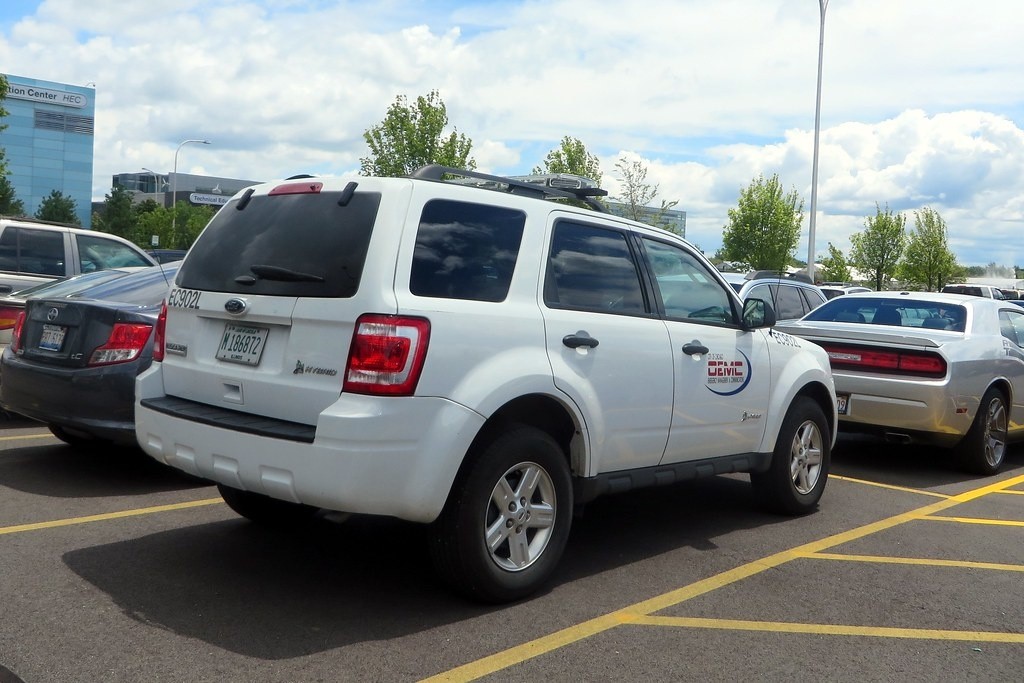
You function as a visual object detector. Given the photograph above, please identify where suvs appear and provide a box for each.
[1001,288,1024,301]
[0,217,160,360]
[130,165,837,600]
[818,282,934,327]
[940,283,1006,303]
[650,270,828,323]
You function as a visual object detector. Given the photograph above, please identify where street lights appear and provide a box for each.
[142,168,157,208]
[171,139,211,245]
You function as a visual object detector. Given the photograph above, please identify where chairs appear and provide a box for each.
[872,308,901,326]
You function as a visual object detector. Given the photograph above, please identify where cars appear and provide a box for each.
[0,260,185,450]
[106,249,188,267]
[774,291,1023,471]
